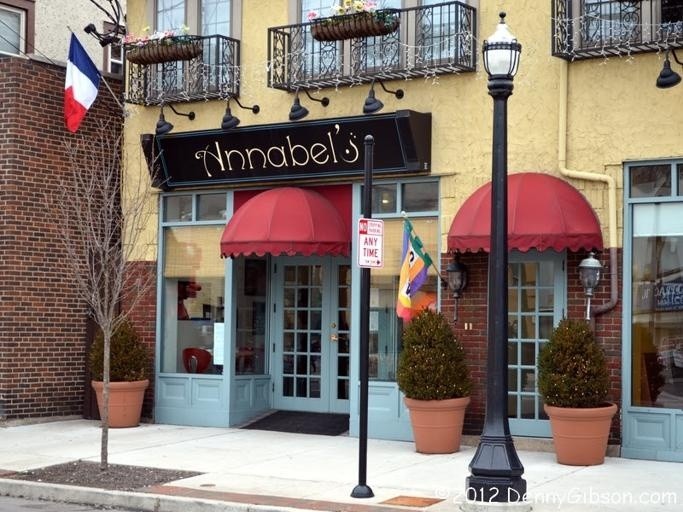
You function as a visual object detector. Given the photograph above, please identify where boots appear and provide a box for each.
[239,293,304,377]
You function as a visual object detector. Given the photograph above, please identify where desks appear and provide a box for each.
[461,10,533,503]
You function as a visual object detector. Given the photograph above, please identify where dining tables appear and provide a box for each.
[397,305,471,455]
[86,316,148,429]
[536,318,617,464]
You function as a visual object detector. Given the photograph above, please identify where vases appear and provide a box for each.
[304,0,385,18]
[120,23,191,47]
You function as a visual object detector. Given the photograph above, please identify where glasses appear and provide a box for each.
[575,252,603,320]
[149,78,404,135]
[654,45,683,91]
[443,256,470,321]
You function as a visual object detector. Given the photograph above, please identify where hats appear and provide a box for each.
[365,326,397,379]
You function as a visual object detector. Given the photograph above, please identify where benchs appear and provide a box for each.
[62,30,101,133]
[394,217,433,323]
